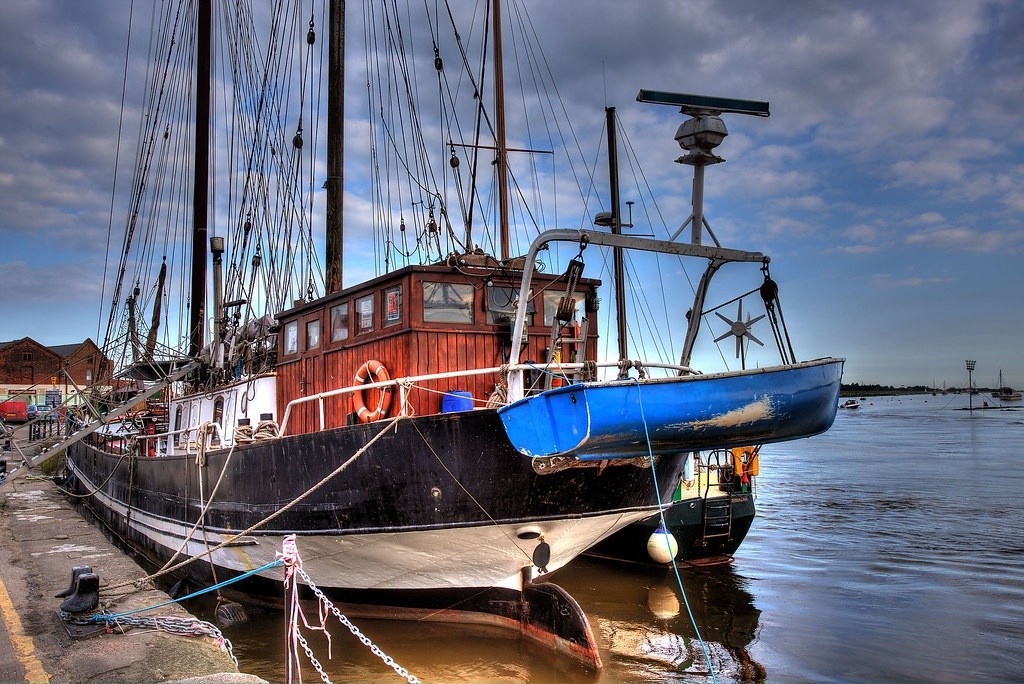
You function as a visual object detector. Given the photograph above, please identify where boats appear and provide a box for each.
[495,354,847,460]
[838,398,862,408]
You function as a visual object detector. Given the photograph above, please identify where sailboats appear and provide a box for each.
[942,378,947,395]
[52,1,849,681]
[567,98,763,577]
[931,378,937,396]
[989,368,1023,401]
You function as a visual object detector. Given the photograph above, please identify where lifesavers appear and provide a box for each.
[353,360,393,423]
[217,601,247,622]
[731,446,758,477]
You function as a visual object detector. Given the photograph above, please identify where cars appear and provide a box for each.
[27,405,59,423]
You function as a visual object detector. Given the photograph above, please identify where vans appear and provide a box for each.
[0,401,28,425]
